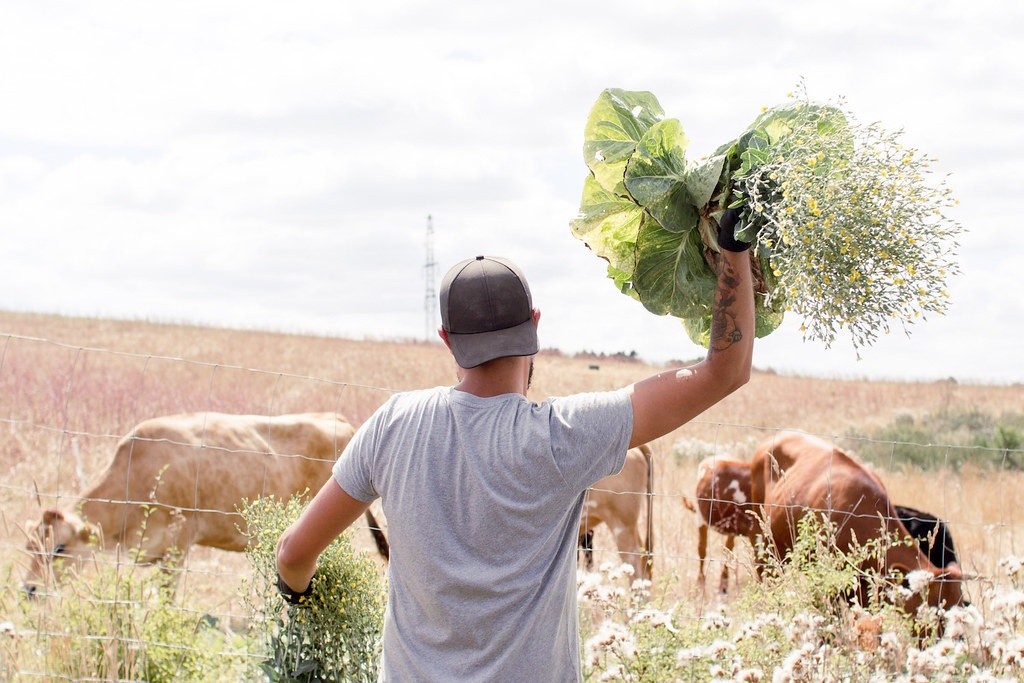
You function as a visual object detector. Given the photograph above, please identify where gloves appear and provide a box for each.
[716,202,752,252]
[277,570,326,610]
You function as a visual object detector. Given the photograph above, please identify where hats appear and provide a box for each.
[439,256,539,369]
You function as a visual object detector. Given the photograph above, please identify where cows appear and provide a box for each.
[682,454,752,592]
[22,412,391,599]
[895,506,970,608]
[578,444,655,583]
[749,427,962,652]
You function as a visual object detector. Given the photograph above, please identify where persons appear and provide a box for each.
[274,203,755,683]
